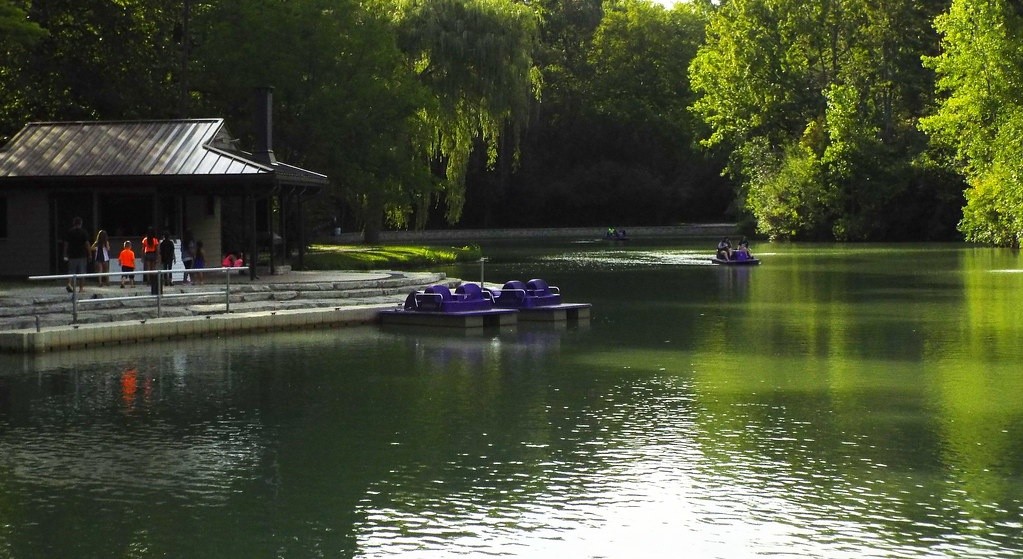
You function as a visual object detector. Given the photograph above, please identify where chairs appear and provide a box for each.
[500,281,537,308]
[717,246,730,259]
[527,279,561,306]
[425,284,464,311]
[455,283,491,310]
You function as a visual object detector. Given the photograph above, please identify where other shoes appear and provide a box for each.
[99,283,103,287]
[200,281,204,285]
[190,282,193,285]
[131,285,136,288]
[66,284,73,292]
[169,282,173,286]
[165,282,168,286]
[182,282,187,286]
[194,282,198,285]
[121,283,125,289]
[79,291,86,294]
[106,282,111,286]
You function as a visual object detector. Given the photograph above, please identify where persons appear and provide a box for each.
[717,237,732,261]
[737,236,748,255]
[142,228,176,286]
[222,248,244,267]
[181,228,206,285]
[91,230,111,287]
[63,217,91,292]
[119,241,136,288]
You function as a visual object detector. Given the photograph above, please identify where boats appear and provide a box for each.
[708,247,762,266]
[603,232,627,241]
[380,278,520,326]
[484,278,591,329]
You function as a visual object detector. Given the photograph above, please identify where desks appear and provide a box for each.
[731,250,747,260]
